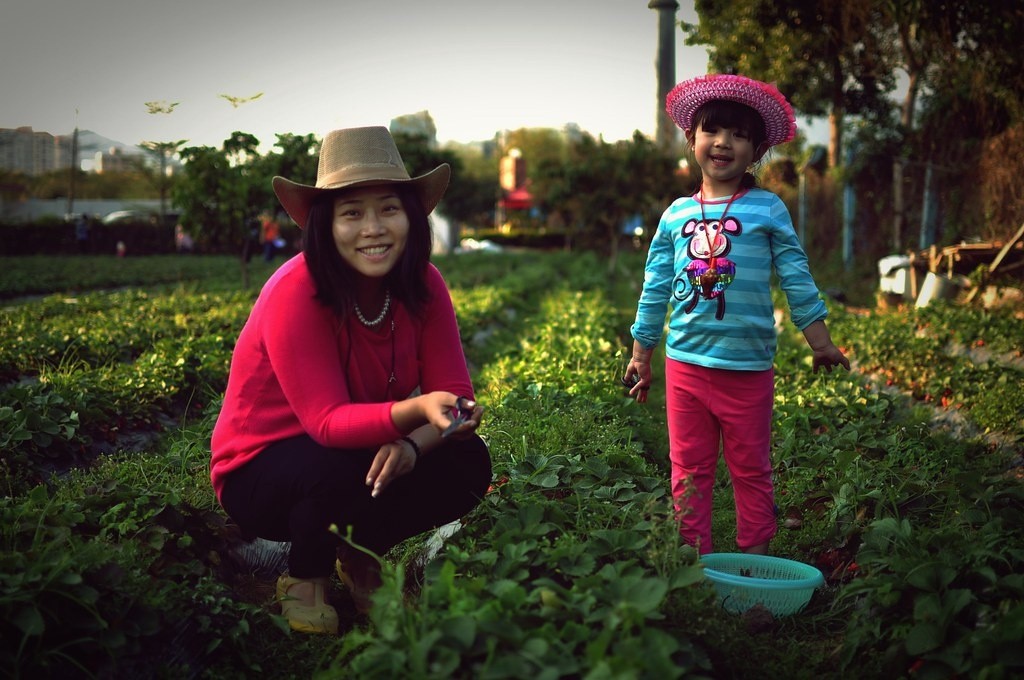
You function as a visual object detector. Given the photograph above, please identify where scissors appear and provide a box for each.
[440,395,475,437]
[621,372,651,390]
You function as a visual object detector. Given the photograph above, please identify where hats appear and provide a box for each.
[667,75,795,147]
[272,126,451,229]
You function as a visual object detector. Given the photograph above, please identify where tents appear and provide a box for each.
[497,178,542,226]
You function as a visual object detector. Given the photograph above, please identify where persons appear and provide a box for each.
[211,125,492,634]
[77,208,279,263]
[624,73,851,557]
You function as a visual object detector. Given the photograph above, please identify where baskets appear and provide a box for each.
[696,553,824,618]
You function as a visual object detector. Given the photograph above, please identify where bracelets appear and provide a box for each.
[400,436,420,461]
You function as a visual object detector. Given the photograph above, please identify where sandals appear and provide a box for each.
[336,556,382,614]
[276,574,338,635]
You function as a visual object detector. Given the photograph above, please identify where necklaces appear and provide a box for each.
[345,287,398,404]
[699,181,742,299]
